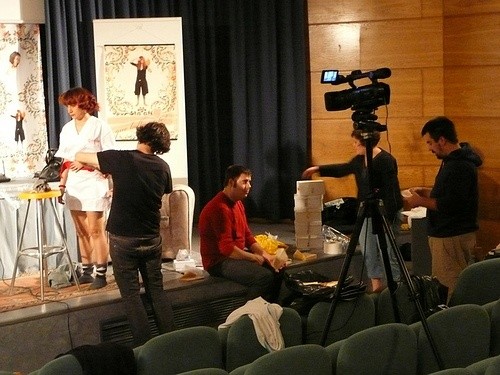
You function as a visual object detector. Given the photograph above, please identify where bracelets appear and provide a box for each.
[58,185,66,188]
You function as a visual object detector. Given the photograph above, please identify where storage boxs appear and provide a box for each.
[294,180,326,249]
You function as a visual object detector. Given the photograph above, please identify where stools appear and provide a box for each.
[8,189,81,301]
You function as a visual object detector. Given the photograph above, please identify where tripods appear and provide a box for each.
[321,209,445,369]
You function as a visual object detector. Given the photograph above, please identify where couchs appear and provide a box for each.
[158,184,195,260]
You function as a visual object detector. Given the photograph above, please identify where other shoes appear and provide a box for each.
[70,275,94,285]
[87,278,107,290]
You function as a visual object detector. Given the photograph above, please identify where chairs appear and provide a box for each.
[33,256,500,375]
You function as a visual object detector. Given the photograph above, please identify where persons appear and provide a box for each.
[75,122,176,348]
[53,87,118,290]
[403,117,483,307]
[301,129,405,293]
[198,164,300,307]
